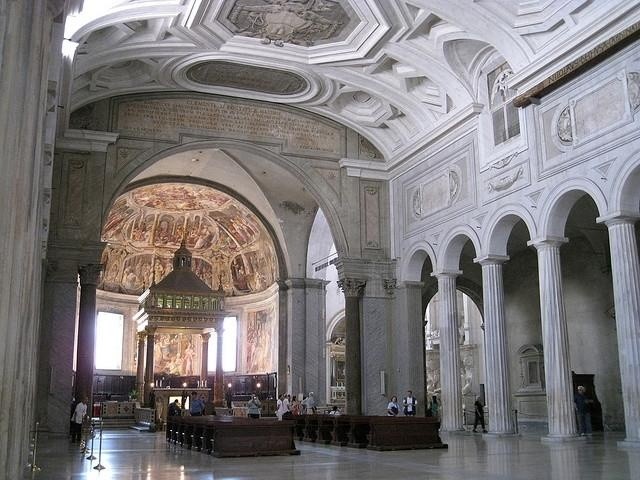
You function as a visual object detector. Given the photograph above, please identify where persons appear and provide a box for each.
[100,198,260,289]
[329,406,340,415]
[387,395,399,416]
[403,390,418,416]
[573,384,593,437]
[473,396,488,433]
[190,391,205,417]
[427,402,434,416]
[169,399,181,415]
[275,391,315,422]
[71,394,91,443]
[248,392,262,418]
[154,333,195,375]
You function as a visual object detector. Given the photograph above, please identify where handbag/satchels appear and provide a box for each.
[388,407,398,415]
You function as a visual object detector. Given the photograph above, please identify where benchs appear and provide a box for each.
[166,414,448,458]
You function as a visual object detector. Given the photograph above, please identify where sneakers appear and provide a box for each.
[473,429,488,433]
